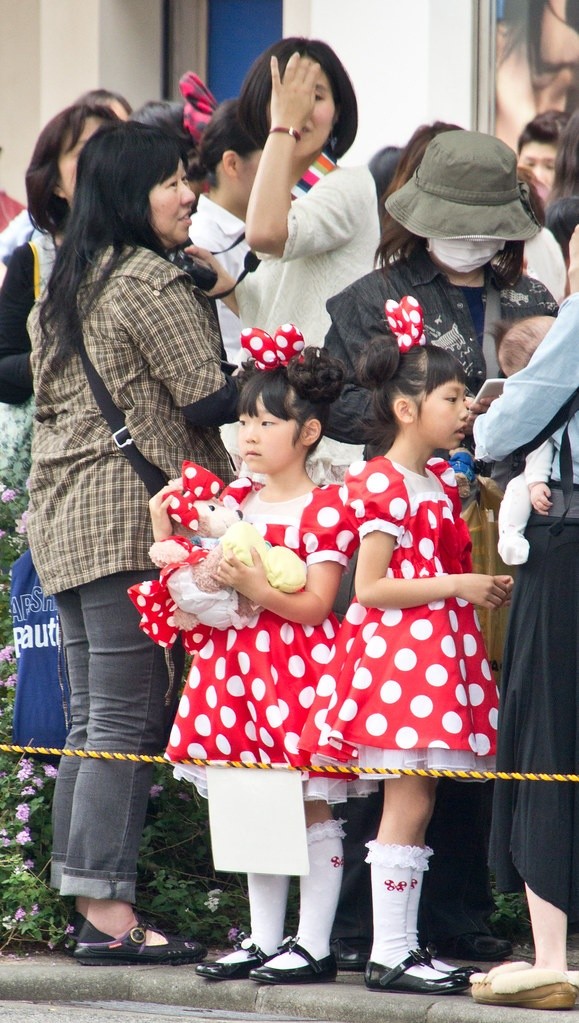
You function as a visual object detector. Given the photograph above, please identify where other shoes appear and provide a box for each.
[469,961,579,1009]
[64,910,208,965]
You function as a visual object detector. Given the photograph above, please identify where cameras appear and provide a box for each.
[164,237,219,292]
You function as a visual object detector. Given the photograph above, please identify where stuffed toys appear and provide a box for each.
[125,458,310,650]
[126,457,307,650]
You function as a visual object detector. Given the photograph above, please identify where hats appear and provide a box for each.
[385,130,540,240]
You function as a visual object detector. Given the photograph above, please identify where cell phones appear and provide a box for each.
[465,379,506,435]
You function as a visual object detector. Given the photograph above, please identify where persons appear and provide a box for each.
[315,129,557,460]
[297,294,515,999]
[0,37,579,1011]
[22,119,244,965]
[182,38,381,354]
[473,110,576,1009]
[162,321,380,986]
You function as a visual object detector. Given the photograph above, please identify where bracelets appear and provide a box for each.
[270,127,301,142]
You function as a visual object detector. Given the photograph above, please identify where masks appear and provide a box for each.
[425,238,502,274]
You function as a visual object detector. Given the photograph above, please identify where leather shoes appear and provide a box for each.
[421,933,512,961]
[195,931,338,984]
[364,942,481,994]
[330,938,371,969]
